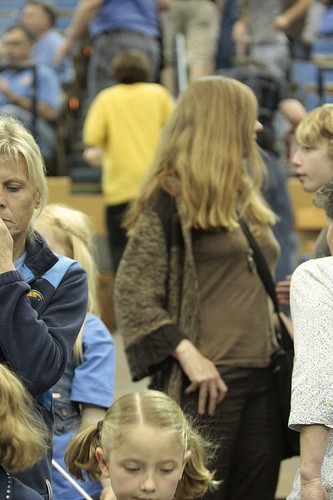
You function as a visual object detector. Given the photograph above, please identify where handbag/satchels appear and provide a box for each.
[269,334,302,457]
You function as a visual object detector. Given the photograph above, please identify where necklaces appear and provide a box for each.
[247,248,256,273]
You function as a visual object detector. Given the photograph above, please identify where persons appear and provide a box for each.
[0,0,333,500]
[113,75,294,500]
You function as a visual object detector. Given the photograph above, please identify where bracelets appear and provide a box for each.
[64,28,77,39]
